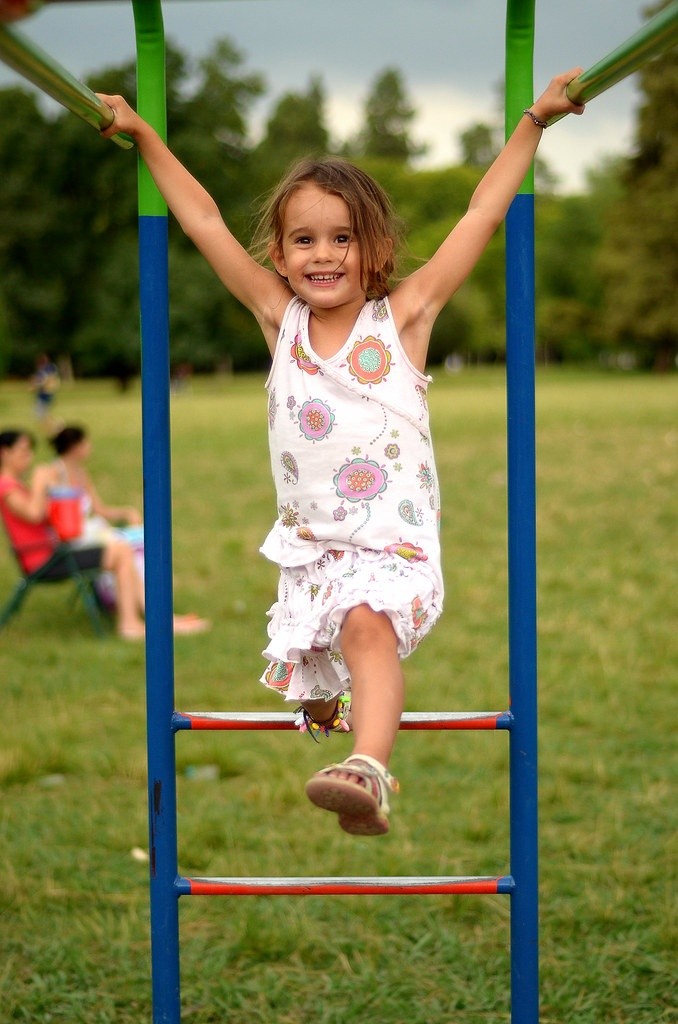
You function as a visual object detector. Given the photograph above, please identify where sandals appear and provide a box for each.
[305,754,399,835]
[305,701,351,733]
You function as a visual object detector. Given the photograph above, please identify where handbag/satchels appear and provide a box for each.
[46,459,83,541]
[97,532,144,605]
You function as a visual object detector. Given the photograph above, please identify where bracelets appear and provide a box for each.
[524,109,547,128]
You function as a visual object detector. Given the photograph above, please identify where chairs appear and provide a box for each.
[0,502,112,633]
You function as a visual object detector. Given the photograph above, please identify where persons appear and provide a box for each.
[95,66,585,838]
[32,356,59,419]
[0,426,211,642]
